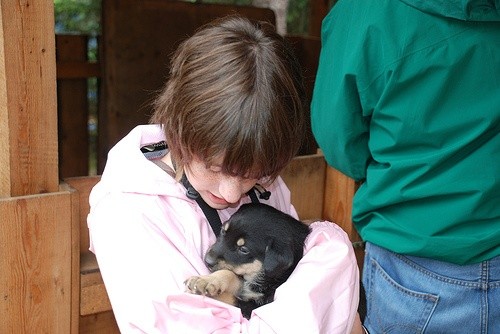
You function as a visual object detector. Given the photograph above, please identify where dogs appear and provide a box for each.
[183,203,368,325]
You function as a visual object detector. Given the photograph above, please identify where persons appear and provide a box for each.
[85,14,366,334]
[307,0,499,333]
